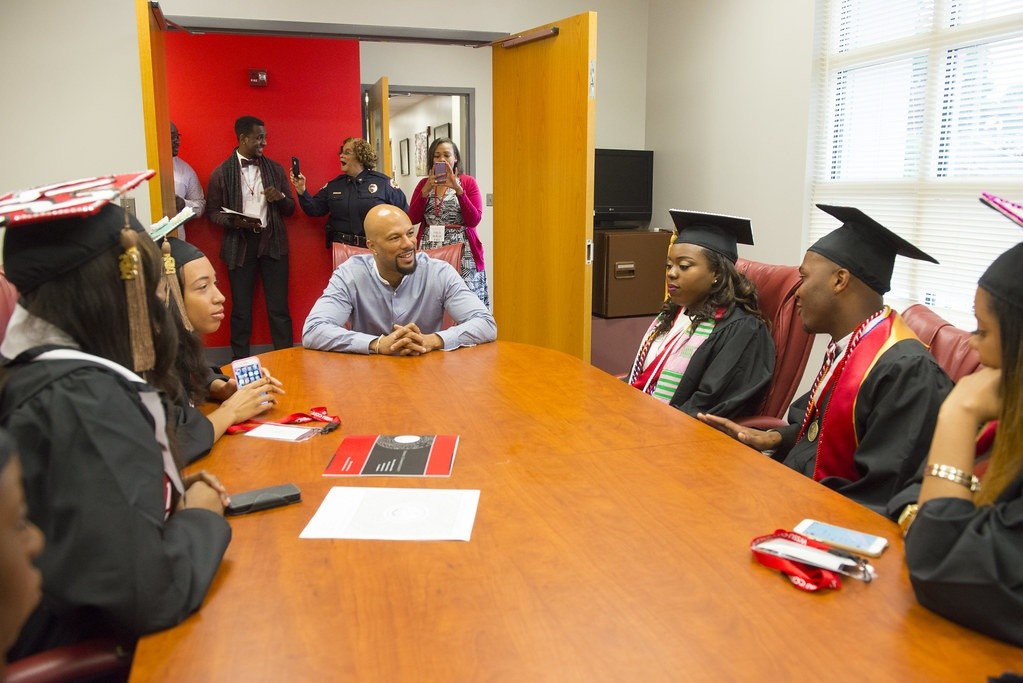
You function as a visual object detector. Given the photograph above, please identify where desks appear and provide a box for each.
[131,339,1023,683]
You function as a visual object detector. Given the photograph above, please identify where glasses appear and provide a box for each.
[171,133,182,140]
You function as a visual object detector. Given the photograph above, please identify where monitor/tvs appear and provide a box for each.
[592,149,654,230]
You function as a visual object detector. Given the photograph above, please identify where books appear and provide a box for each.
[323,435,461,477]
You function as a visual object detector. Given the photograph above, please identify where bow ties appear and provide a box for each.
[241,159,260,168]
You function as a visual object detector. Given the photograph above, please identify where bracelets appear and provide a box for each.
[375,335,384,354]
[925,463,980,493]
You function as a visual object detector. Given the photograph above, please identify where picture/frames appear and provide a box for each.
[400,137,410,175]
[434,123,450,141]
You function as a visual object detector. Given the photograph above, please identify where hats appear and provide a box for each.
[806,204,940,296]
[664,209,754,304]
[977,193,1023,310]
[0,170,157,372]
[147,206,196,333]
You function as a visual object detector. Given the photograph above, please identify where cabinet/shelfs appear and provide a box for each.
[592,228,675,318]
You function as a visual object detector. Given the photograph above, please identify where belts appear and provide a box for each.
[247,227,264,234]
[333,232,366,248]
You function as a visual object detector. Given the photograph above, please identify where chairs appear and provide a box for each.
[734,259,986,420]
[331,241,465,334]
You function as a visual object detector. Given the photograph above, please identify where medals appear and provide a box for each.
[808,422,818,442]
[898,504,917,536]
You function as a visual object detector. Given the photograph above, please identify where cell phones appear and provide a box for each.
[435,162,446,185]
[292,157,300,179]
[230,356,268,405]
[225,484,301,515]
[794,518,888,557]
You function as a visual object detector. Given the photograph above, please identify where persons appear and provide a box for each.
[301,204,498,356]
[0,433,44,658]
[887,242,1023,647]
[624,210,776,417]
[289,137,409,251]
[170,122,206,241]
[697,203,956,517]
[145,207,285,464]
[206,115,296,357]
[407,137,491,312]
[0,171,233,649]
